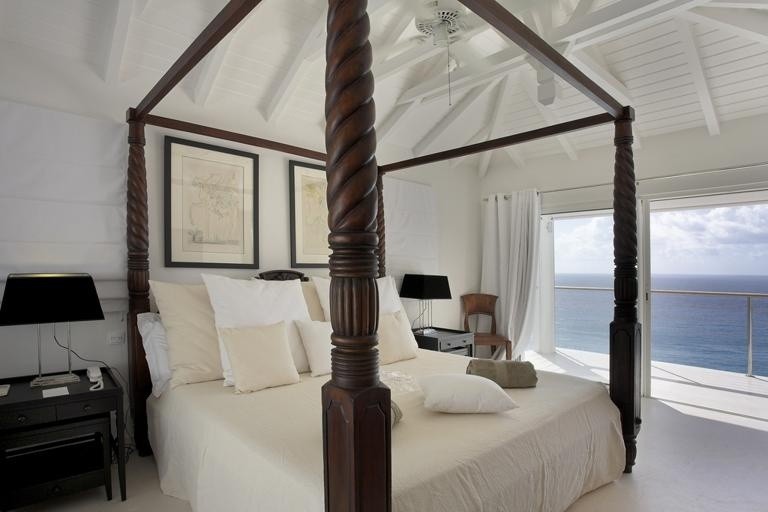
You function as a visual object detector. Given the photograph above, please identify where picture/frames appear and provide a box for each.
[287,160,332,269]
[161,135,259,269]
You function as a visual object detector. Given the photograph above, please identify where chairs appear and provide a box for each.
[461,291,512,362]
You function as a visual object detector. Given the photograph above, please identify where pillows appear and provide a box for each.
[135,277,421,398]
[391,398,404,429]
[419,371,520,414]
[466,355,538,390]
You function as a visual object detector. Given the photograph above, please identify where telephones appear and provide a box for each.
[87,366,103,383]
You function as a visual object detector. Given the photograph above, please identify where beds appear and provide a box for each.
[126,2,644,511]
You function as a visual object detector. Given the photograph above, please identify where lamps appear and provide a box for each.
[0,271,106,388]
[399,273,451,336]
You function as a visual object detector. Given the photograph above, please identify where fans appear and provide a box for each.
[367,0,502,110]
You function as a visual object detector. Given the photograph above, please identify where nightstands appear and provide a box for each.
[0,365,128,504]
[411,326,474,358]
